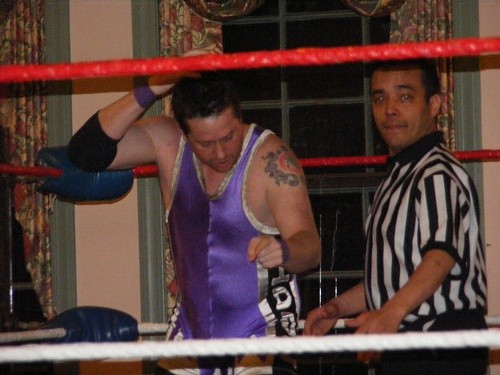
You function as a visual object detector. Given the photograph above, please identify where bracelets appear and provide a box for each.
[279,240,291,265]
[132,84,157,110]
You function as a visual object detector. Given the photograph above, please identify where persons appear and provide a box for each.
[298,58,492,375]
[66,46,322,375]
